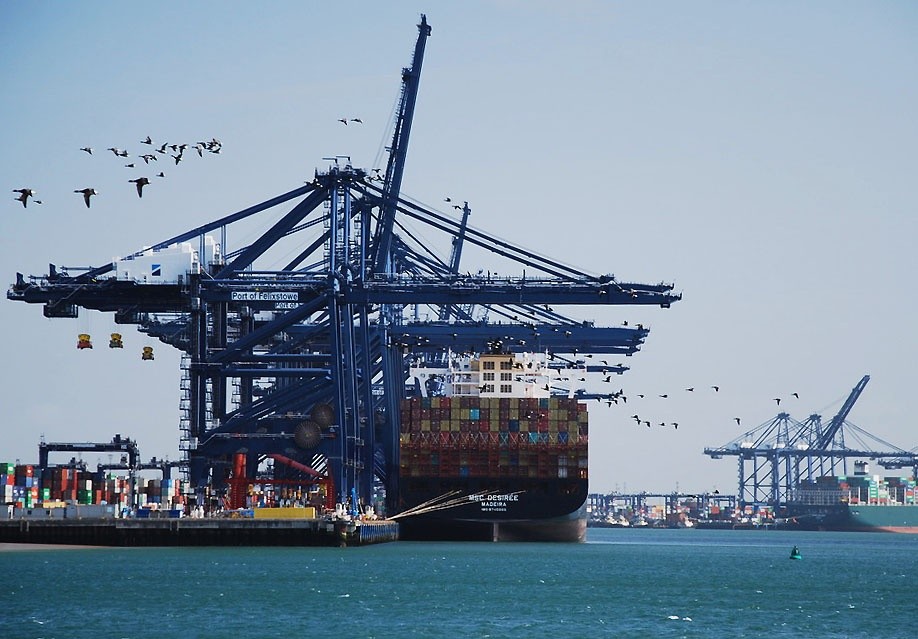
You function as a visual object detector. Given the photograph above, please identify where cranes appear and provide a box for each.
[702,372,918,522]
[5,10,684,524]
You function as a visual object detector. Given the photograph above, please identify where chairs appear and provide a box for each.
[49,264,67,280]
[16,271,35,288]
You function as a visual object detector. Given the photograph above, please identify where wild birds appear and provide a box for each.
[179,144,188,155]
[206,138,222,154]
[128,177,150,198]
[366,167,800,429]
[351,118,362,124]
[80,147,92,154]
[192,144,204,157]
[74,188,96,210]
[155,142,168,154]
[140,136,151,144]
[197,142,207,149]
[107,148,128,157]
[156,171,164,177]
[168,144,178,151]
[145,154,157,161]
[13,188,42,209]
[171,154,182,165]
[138,155,148,164]
[338,119,347,125]
[125,163,134,167]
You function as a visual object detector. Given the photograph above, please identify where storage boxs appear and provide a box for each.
[589,475,918,529]
[399,395,588,480]
[0,456,197,520]
